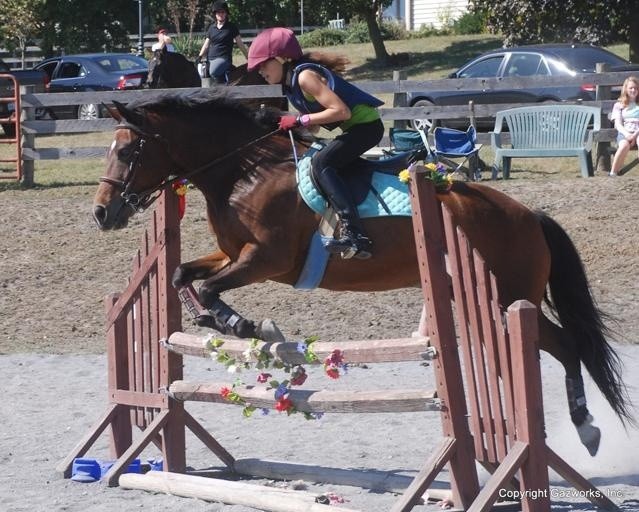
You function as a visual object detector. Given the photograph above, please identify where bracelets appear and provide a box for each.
[301,114,310,127]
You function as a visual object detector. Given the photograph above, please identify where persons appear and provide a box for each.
[195,0,248,85]
[247,27,385,258]
[610,76,639,177]
[152,28,175,52]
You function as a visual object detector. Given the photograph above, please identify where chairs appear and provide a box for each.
[381,127,427,163]
[430,125,483,183]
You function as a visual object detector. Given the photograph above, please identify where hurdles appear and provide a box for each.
[57,166,621,511]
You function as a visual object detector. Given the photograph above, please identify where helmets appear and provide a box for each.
[247,28,302,72]
[212,1,229,13]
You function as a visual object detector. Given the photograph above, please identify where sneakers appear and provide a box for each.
[324,226,373,259]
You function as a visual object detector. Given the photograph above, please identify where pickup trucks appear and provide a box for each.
[0,57,52,138]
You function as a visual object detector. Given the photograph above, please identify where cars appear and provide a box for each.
[405,40,639,137]
[26,52,154,120]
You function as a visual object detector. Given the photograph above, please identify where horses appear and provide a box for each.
[142,45,345,109]
[93,88,635,456]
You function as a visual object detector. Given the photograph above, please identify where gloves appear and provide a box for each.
[196,55,202,64]
[280,115,301,129]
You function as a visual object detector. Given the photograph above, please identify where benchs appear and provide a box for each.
[487,105,601,179]
[607,145,639,176]
